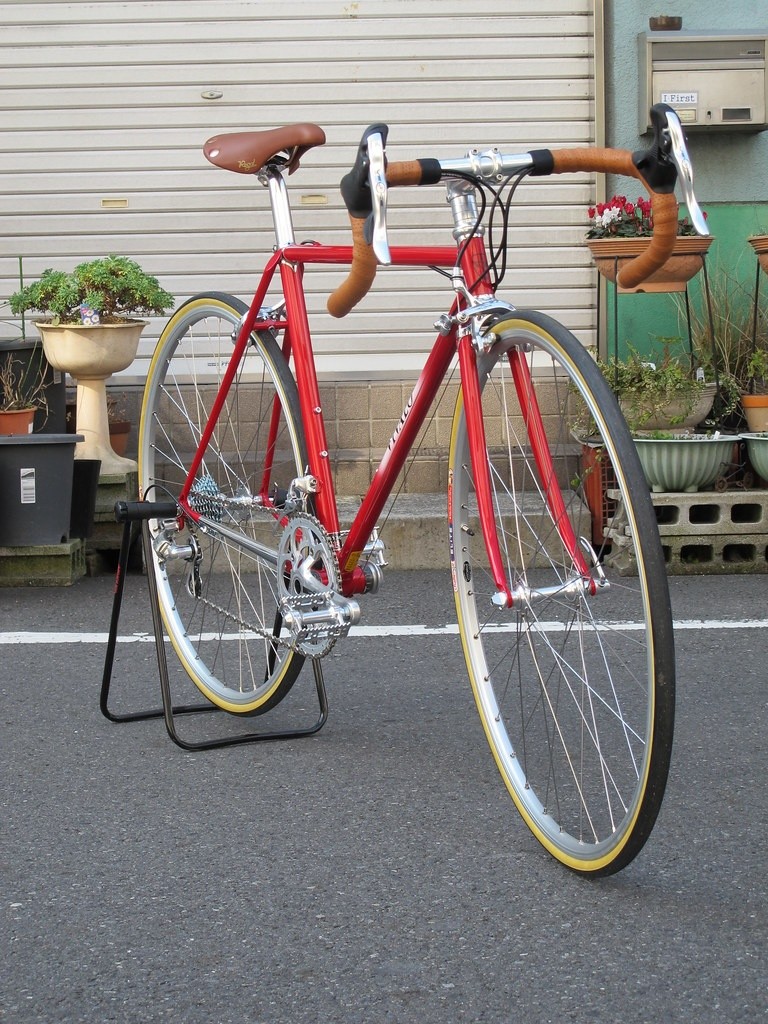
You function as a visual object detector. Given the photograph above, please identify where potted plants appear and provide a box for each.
[738,433,768,483]
[4,253,176,380]
[632,431,743,493]
[0,339,56,434]
[740,349,768,433]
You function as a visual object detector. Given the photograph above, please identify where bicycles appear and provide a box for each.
[99,102,711,878]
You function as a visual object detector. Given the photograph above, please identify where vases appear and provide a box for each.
[108,419,130,457]
[69,459,102,540]
[584,237,717,293]
[612,380,723,436]
[65,401,118,434]
[746,235,768,274]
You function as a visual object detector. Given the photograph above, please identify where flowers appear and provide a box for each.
[584,197,708,240]
[558,337,741,487]
